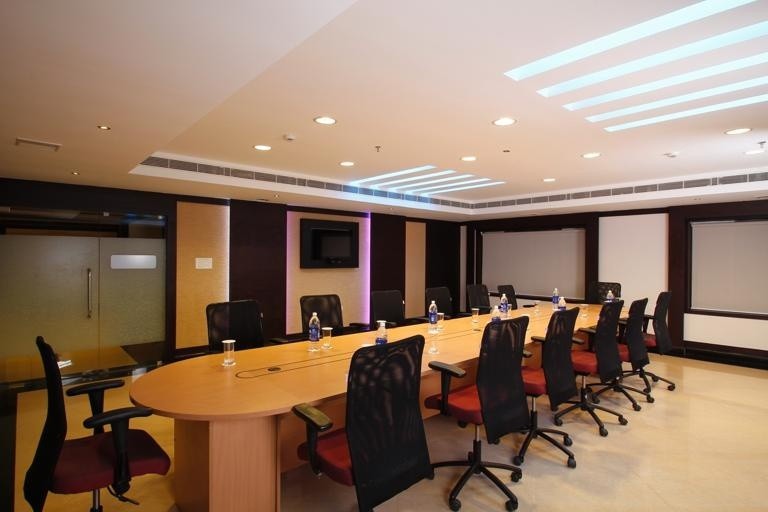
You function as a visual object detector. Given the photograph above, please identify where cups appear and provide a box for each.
[428,325,439,355]
[471,308,479,322]
[438,313,444,329]
[322,327,331,349]
[223,340,235,366]
[535,303,541,316]
[582,304,588,320]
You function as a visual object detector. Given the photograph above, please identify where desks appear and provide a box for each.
[130,301,639,512]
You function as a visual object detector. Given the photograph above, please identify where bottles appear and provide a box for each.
[375,322,388,346]
[307,312,321,353]
[606,289,615,303]
[500,293,508,318]
[428,300,438,335]
[552,288,559,310]
[558,297,566,311]
[491,305,501,322]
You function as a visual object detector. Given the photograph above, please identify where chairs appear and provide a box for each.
[428,286,474,322]
[208,298,291,351]
[513,307,581,468]
[425,316,533,512]
[577,297,677,394]
[371,290,428,330]
[24,337,170,511]
[466,283,502,315]
[497,285,538,313]
[290,334,435,512]
[532,298,629,438]
[299,293,369,339]
[624,291,675,355]
[571,297,655,411]
[584,282,622,305]
